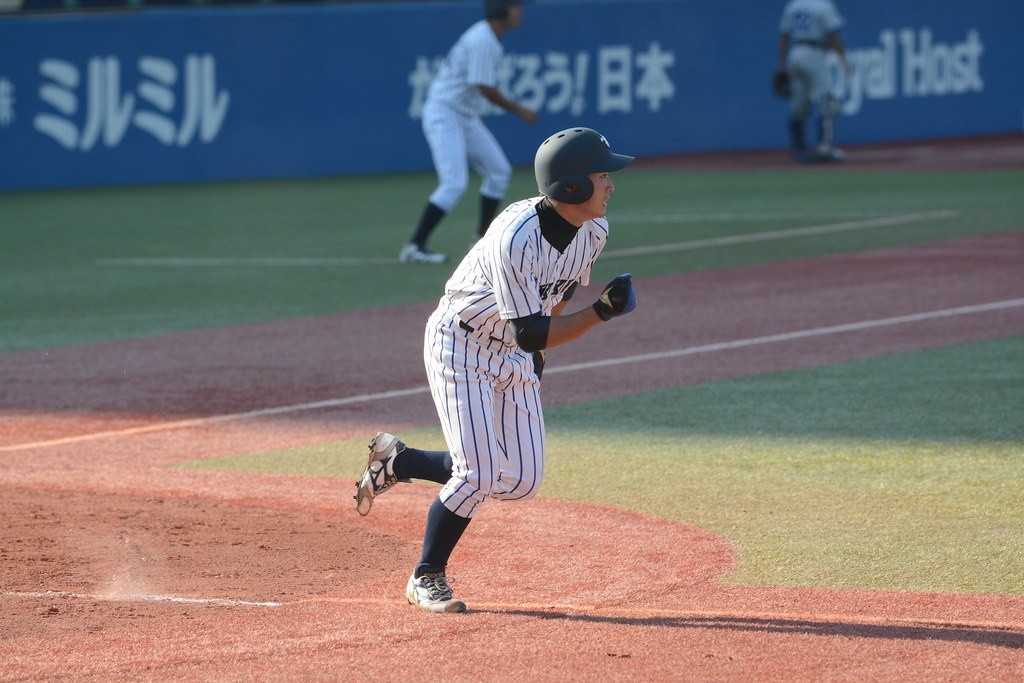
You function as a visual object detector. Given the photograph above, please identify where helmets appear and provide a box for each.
[534,127,636,204]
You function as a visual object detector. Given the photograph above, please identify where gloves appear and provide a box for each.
[592,273,636,322]
[532,350,546,380]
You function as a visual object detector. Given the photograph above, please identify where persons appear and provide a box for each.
[398,1,536,265]
[776,0,852,160]
[354,127,636,615]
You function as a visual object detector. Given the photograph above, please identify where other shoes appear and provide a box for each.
[399,245,447,262]
[817,143,845,161]
[791,147,814,163]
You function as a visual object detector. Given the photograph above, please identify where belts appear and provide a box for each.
[459,321,501,341]
[795,39,825,48]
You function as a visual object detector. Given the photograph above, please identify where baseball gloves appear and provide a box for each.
[774,72,792,95]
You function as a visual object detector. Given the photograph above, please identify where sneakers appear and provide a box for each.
[354,432,413,517]
[406,570,466,613]
